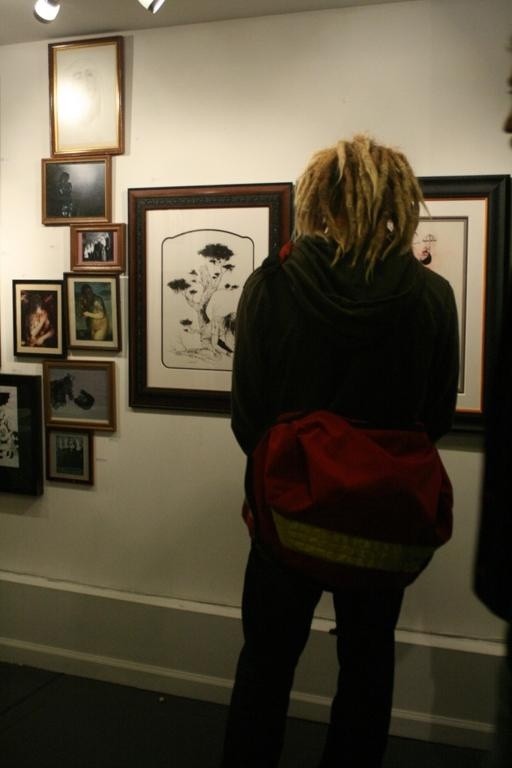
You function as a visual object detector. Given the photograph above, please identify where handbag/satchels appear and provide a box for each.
[241,402,459,589]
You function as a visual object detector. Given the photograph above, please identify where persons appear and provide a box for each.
[56,172,72,218]
[470,43,512,768]
[25,304,55,347]
[222,136,459,767]
[79,284,108,341]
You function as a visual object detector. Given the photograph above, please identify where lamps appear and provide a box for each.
[32,0,61,24]
[138,0,165,14]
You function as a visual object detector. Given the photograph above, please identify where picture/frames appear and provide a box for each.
[42,359,117,431]
[0,372,43,495]
[45,428,93,485]
[388,174,512,437]
[48,35,125,157]
[128,182,293,415]
[69,224,126,271]
[63,272,122,352]
[41,157,112,225]
[12,279,67,358]
